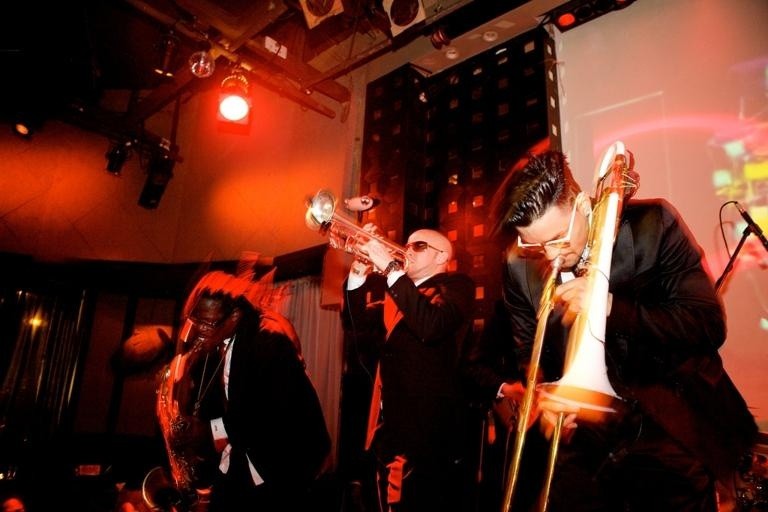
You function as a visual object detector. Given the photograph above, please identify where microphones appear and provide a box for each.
[733,200,768,250]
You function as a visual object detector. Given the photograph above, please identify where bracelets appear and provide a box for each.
[381,260,400,276]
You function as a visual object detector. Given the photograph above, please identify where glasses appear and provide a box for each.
[518,191,583,252]
[404,240,445,253]
[188,314,232,329]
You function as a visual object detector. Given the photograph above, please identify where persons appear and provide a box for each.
[340,220,486,511]
[495,149,762,511]
[465,297,535,512]
[153,269,332,512]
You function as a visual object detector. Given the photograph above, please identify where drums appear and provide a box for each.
[731,433,768,511]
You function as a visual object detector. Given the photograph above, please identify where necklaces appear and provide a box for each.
[192,335,232,416]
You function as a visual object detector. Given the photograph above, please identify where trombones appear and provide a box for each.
[501,142,639,511]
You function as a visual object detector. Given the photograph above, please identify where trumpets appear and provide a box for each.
[305,189,409,276]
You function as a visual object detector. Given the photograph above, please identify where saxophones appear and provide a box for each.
[141,337,206,511]
